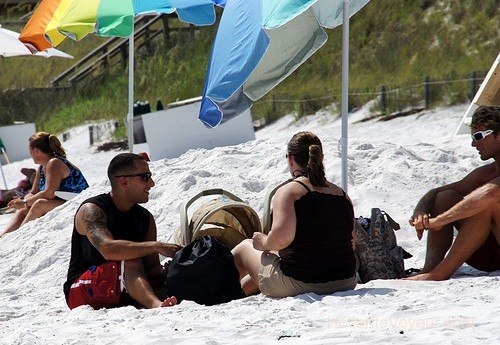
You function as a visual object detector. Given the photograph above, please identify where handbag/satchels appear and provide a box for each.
[165,234,246,306]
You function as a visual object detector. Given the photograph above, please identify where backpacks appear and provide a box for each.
[353,208,413,285]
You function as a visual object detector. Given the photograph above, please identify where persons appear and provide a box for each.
[400,105,500,282]
[230,132,357,297]
[63,152,184,310]
[0,132,89,239]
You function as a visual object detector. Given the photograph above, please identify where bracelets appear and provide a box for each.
[23,199,27,207]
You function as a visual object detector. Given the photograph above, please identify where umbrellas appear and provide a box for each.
[0,28,74,59]
[18,0,228,153]
[199,0,371,192]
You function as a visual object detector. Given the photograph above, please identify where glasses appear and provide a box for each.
[471,129,493,142]
[114,173,151,181]
[286,154,293,158]
